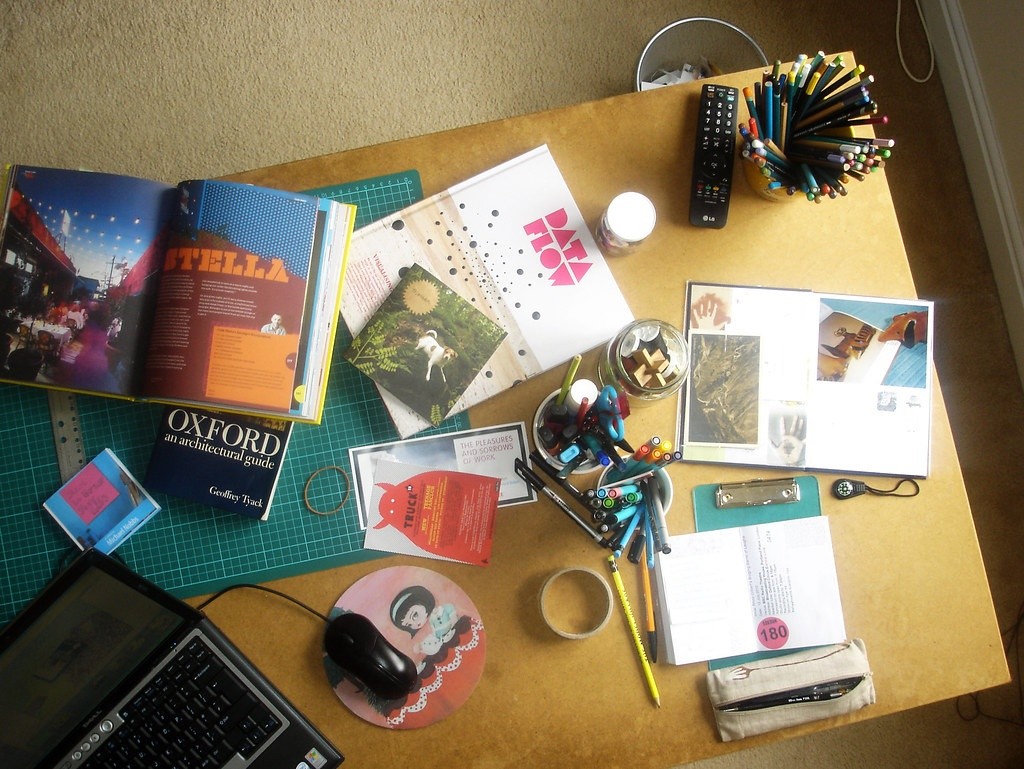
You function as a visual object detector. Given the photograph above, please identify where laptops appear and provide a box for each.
[0,547,344,768]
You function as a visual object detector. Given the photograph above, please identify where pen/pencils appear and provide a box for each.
[736,50,896,206]
[636,536,660,665]
[512,457,611,551]
[529,449,601,519]
[534,354,681,559]
[606,555,664,709]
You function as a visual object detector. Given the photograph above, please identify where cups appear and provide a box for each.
[596,455,673,530]
[742,89,855,202]
[599,318,688,405]
[532,383,619,473]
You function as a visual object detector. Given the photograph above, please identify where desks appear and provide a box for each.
[1,48,1013,767]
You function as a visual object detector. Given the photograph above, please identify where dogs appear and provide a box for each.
[413,329,458,383]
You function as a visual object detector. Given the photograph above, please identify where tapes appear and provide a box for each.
[536,565,614,640]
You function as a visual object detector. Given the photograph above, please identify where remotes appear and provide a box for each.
[686,82,740,230]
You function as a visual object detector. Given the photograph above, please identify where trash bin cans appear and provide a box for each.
[632,17,770,91]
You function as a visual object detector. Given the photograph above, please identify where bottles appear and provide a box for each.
[595,192,658,259]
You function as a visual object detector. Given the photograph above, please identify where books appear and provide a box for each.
[0,159,357,424]
[675,279,936,481]
[143,396,296,523]
[341,262,509,425]
[42,446,163,558]
[654,513,847,667]
[339,143,638,439]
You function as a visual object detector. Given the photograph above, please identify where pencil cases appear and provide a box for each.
[706,638,877,741]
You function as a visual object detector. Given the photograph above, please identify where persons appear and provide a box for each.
[261,312,287,335]
[26,288,100,326]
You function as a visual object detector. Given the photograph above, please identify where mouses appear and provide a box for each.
[326,611,418,701]
